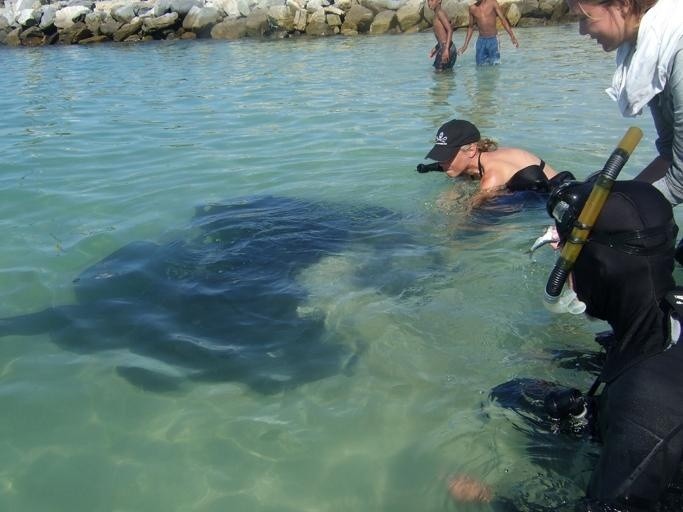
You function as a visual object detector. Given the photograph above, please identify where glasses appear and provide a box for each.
[547,170,676,257]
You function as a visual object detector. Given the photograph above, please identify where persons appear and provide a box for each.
[418,119,575,214]
[446,171,683,511]
[550,0,682,251]
[457,0,519,65]
[428,0,457,73]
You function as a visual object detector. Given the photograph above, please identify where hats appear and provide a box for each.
[426,118,480,162]
